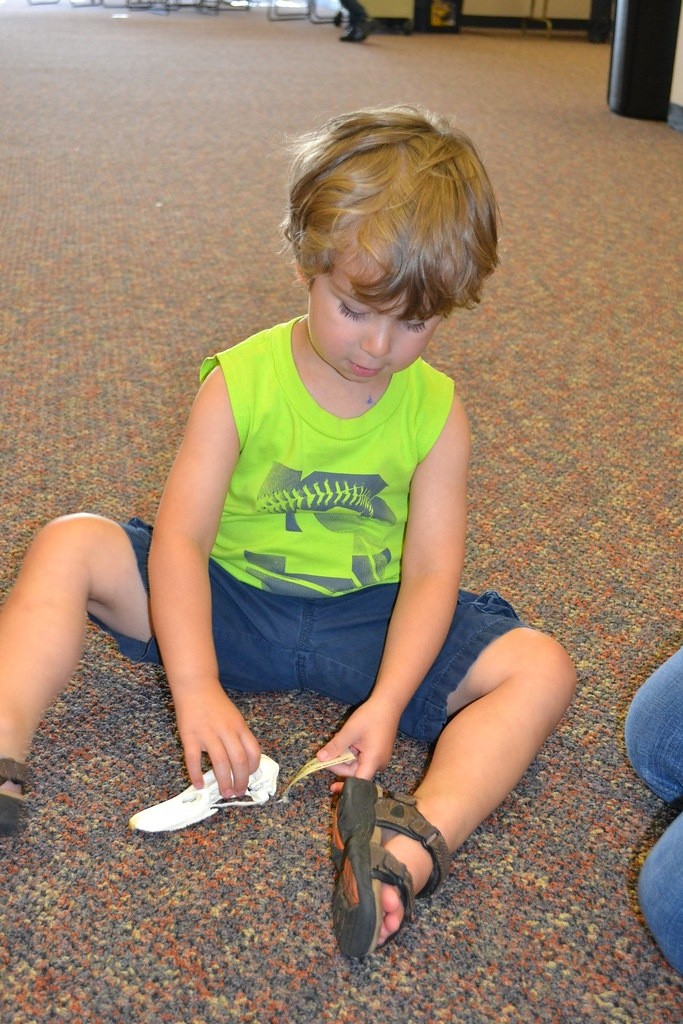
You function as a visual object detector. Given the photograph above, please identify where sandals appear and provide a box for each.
[0,757,27,832]
[330,776,451,958]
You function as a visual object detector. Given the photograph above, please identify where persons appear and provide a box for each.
[2,103,576,958]
[625,642,683,973]
